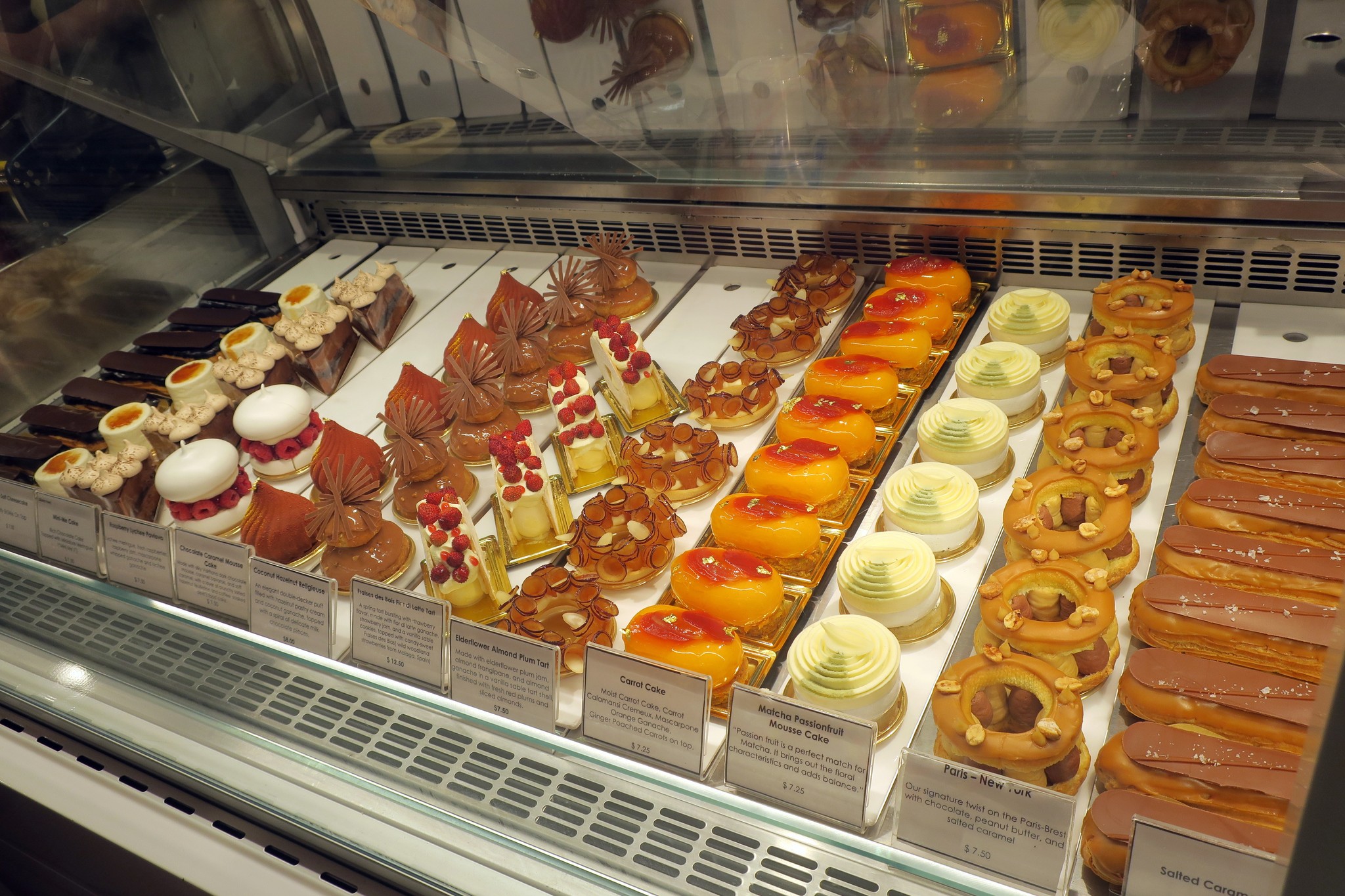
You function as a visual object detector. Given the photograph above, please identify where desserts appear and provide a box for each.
[0,231,1345,883]
[361,1,1258,185]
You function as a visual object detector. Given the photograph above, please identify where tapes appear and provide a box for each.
[370,116,463,168]
